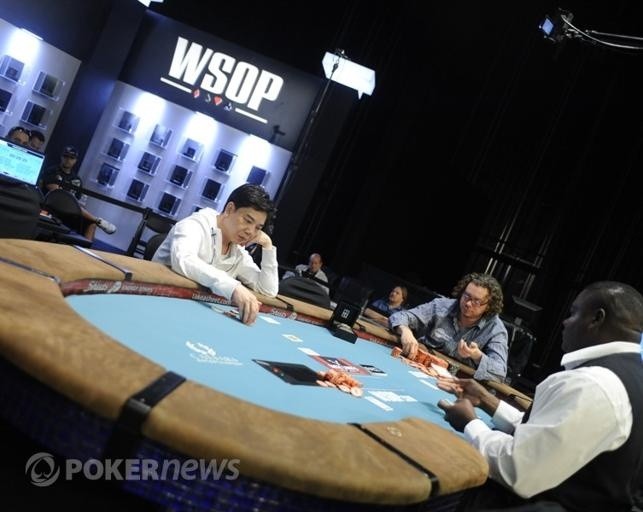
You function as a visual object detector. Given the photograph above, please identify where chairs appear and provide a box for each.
[277,276,336,310]
[141,233,168,261]
[125,207,172,257]
[0,170,98,248]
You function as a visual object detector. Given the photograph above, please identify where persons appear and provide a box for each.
[389,273,509,381]
[151,183,280,324]
[437,281,643,512]
[372,286,408,315]
[28,130,45,151]
[42,145,117,242]
[5,126,30,146]
[282,253,330,296]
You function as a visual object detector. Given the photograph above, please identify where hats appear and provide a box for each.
[62,144,80,158]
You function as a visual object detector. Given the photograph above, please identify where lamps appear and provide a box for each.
[249,49,377,254]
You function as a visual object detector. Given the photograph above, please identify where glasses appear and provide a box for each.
[461,293,489,307]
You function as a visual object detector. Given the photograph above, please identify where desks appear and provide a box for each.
[0,239,533,512]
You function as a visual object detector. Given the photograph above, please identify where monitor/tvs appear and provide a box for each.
[0,136,45,186]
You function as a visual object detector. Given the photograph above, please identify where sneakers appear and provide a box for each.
[95,216,117,234]
